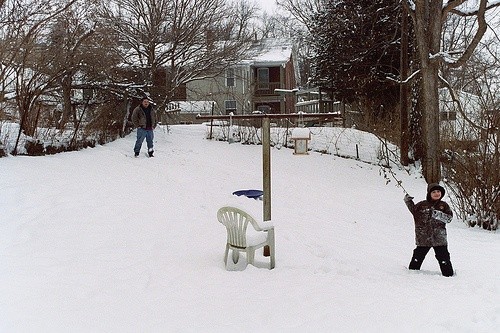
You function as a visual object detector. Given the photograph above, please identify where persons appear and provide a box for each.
[404,183,453,277]
[131,98,158,158]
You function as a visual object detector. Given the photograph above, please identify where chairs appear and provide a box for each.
[217,206,275,270]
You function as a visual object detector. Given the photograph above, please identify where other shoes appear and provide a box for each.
[148,151,155,157]
[134,153,139,156]
